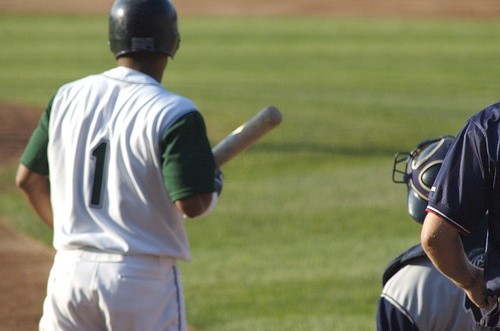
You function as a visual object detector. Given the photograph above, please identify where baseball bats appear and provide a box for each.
[210,104,283,168]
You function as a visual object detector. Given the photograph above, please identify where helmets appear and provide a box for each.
[405,136,458,224]
[108,0,181,60]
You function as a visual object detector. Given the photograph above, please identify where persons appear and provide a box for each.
[15,0,224,331]
[418,103,500,331]
[380,252,476,331]
